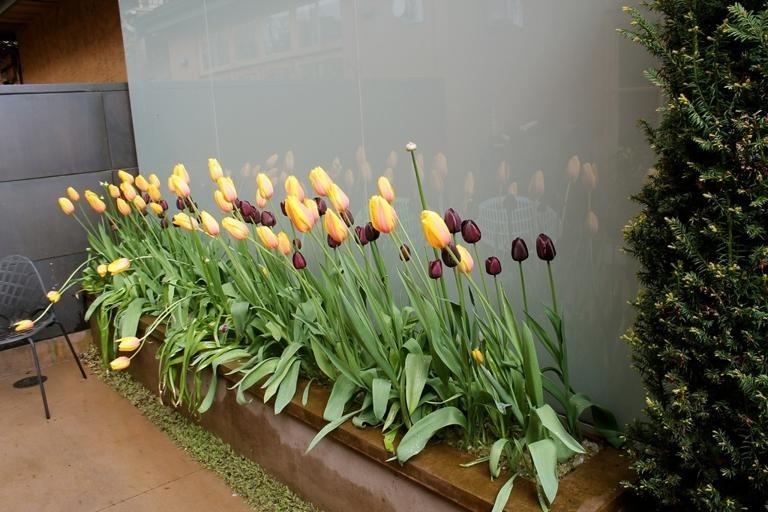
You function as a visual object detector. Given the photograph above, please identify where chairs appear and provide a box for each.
[0,255,87,420]
